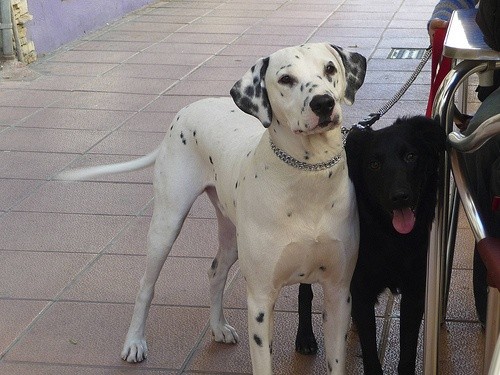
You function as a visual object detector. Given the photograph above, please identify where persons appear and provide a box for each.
[458,0,500,341]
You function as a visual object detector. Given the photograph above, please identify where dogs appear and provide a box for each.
[57,43,367,375]
[294,115,439,375]
